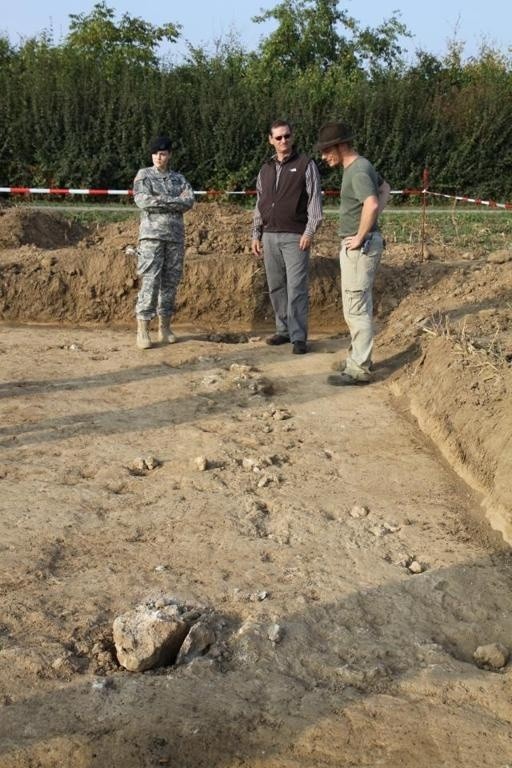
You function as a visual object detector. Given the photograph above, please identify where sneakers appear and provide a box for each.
[293,340,306,354]
[267,335,289,345]
[328,372,369,386]
[332,360,346,371]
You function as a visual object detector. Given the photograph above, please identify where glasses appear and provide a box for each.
[272,134,291,140]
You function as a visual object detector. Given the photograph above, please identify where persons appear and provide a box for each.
[133,137,194,350]
[250,119,324,355]
[314,121,392,386]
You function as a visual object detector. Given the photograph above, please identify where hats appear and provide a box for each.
[315,122,360,150]
[150,136,171,153]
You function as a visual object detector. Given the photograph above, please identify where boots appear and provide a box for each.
[137,320,153,349]
[159,315,178,343]
